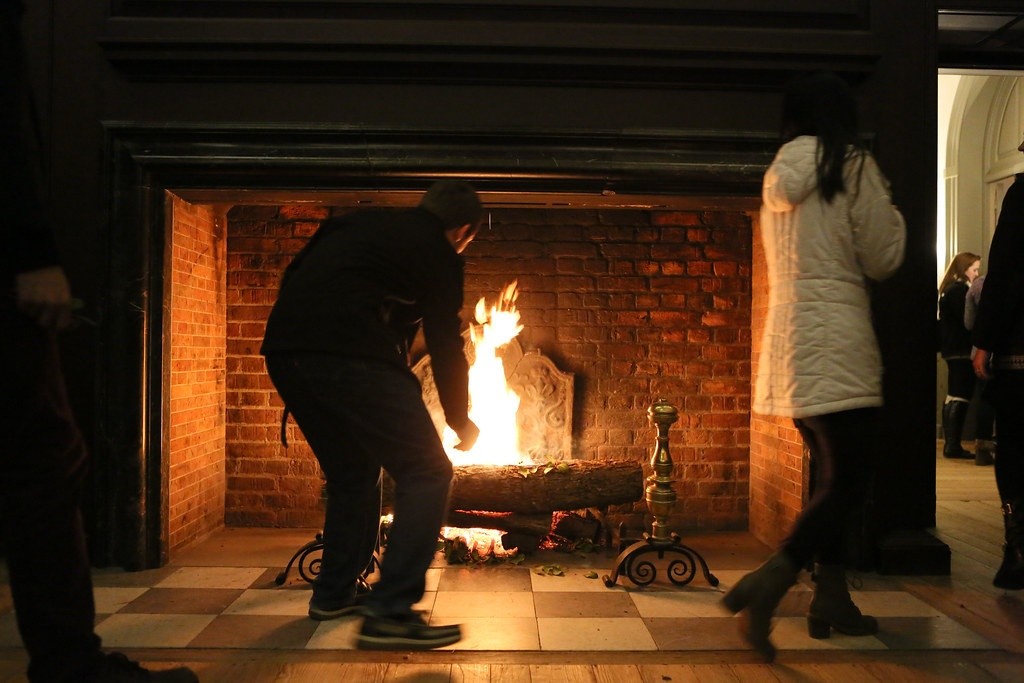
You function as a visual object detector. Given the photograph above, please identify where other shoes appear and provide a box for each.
[353,611,461,651]
[309,580,371,621]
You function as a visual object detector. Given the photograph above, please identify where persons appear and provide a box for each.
[2,0,199,683]
[937,251,981,461]
[259,179,483,653]
[971,136,1024,590]
[718,68,908,663]
[964,253,994,466]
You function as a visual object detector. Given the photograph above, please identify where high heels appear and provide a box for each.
[721,551,800,663]
[808,559,879,640]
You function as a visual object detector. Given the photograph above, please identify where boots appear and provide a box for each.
[975,439,996,466]
[993,497,1024,589]
[942,395,976,460]
